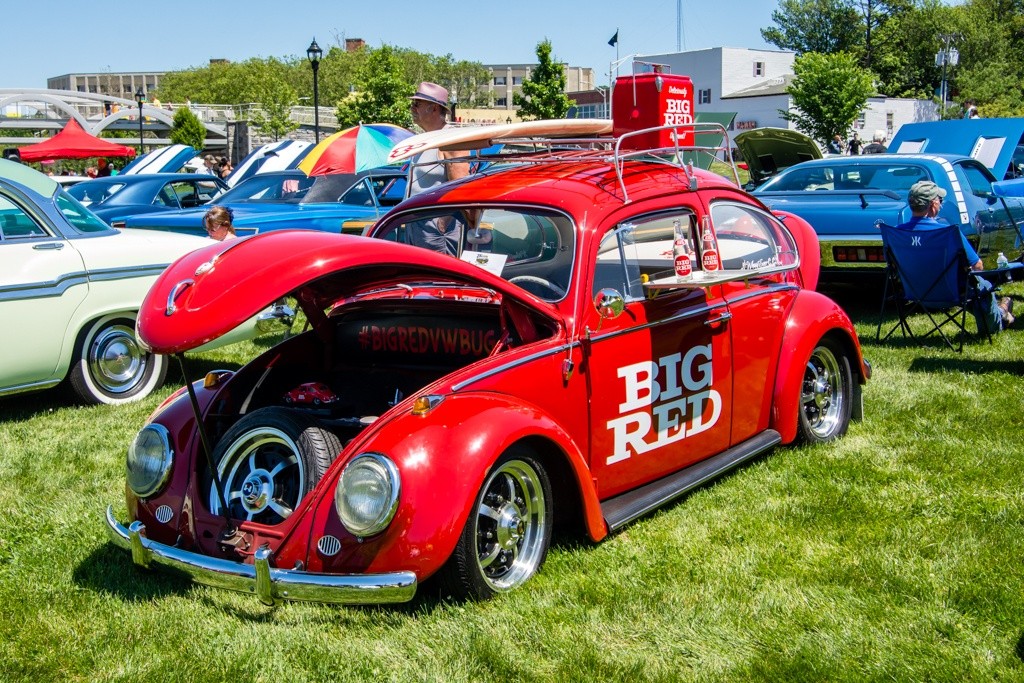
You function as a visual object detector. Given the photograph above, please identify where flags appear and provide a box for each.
[608,31,618,47]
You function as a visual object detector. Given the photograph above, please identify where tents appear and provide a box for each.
[18,118,136,167]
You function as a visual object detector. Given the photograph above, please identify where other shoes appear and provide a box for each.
[998,296,1013,331]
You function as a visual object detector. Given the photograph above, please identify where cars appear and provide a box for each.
[741,117,1024,274]
[0,140,319,238]
[108,170,411,238]
[733,127,862,191]
[104,66,872,603]
[0,175,122,215]
[0,159,277,407]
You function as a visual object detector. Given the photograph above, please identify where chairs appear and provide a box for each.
[875,222,1024,353]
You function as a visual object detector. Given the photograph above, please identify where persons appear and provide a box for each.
[184,95,191,113]
[860,129,888,185]
[194,155,234,199]
[283,176,317,196]
[104,96,173,121]
[829,135,845,154]
[893,180,1016,337]
[963,98,980,119]
[224,106,235,120]
[200,206,240,245]
[846,133,864,156]
[32,157,125,179]
[401,82,471,257]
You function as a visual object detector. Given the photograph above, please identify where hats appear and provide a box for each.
[908,181,947,205]
[408,82,452,114]
[205,155,227,164]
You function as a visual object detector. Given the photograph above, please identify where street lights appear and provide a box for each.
[135,87,145,155]
[306,37,323,144]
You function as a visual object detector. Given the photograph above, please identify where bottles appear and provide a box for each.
[996,252,1013,284]
[700,214,719,279]
[672,218,693,282]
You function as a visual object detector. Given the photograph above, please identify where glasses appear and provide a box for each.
[411,101,436,109]
[203,224,222,232]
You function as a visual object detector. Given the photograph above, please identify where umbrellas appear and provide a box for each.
[299,121,416,204]
